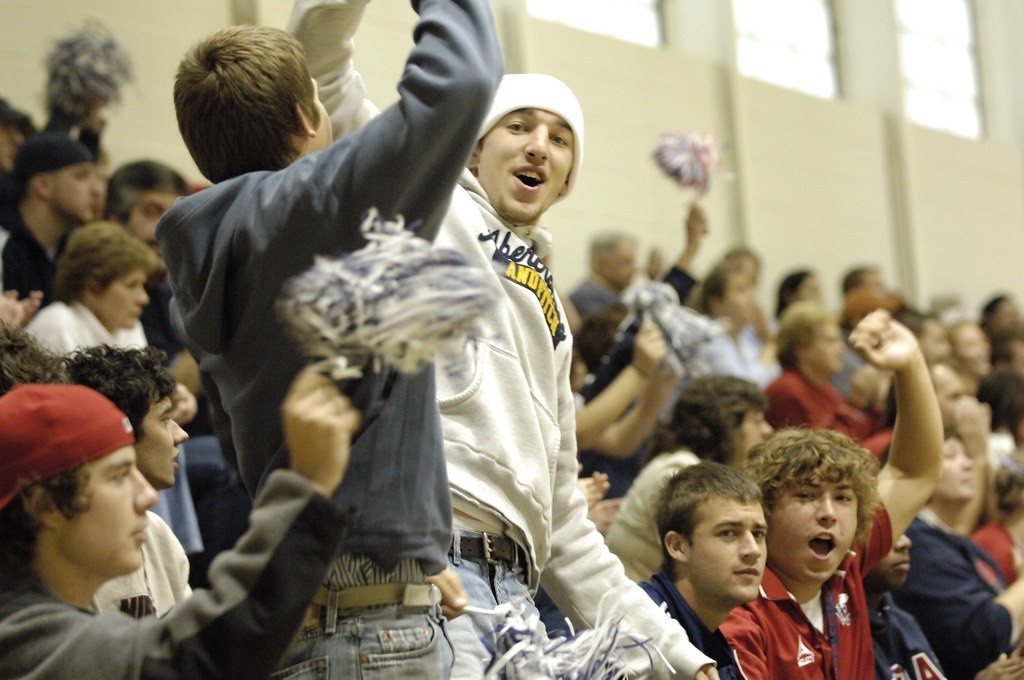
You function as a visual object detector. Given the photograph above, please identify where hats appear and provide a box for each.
[13,132,93,185]
[477,73,584,201]
[0,383,135,510]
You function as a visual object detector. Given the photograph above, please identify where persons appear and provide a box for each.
[634,460,769,680]
[718,308,948,680]
[288,0,721,680]
[1,86,1024,586]
[153,0,506,678]
[862,532,1022,680]
[893,400,1024,678]
[3,356,359,680]
[60,344,196,626]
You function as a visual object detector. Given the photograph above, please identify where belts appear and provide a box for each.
[447,535,529,573]
